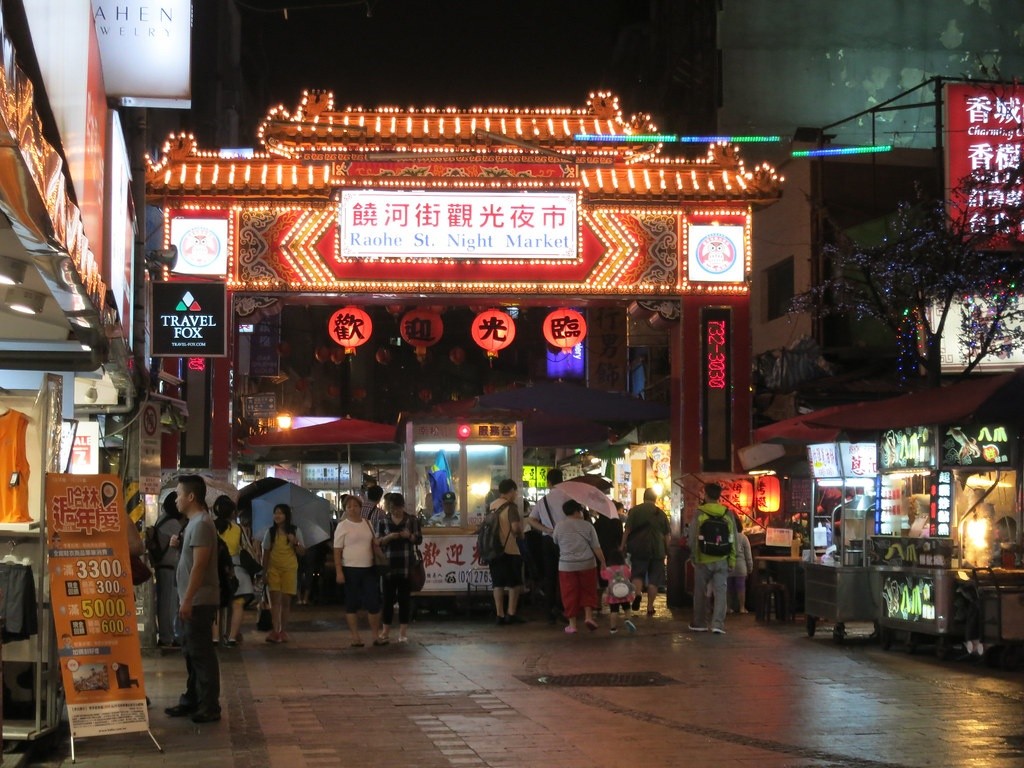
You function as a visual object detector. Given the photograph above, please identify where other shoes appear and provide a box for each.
[548,611,569,625]
[624,618,636,633]
[711,625,724,633]
[266,631,288,643]
[688,622,708,631]
[646,606,656,614]
[739,607,746,615]
[192,709,221,722]
[164,705,194,716]
[226,639,236,648]
[564,626,577,634]
[374,638,388,646]
[632,593,642,611]
[585,619,598,631]
[212,639,219,648]
[398,637,408,643]
[610,627,618,634]
[496,612,528,625]
[352,638,365,646]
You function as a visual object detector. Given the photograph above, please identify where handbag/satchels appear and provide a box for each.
[240,550,262,574]
[410,559,426,592]
[131,557,152,586]
[256,609,272,630]
[627,521,665,558]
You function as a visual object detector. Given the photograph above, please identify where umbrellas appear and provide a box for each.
[555,481,621,522]
[569,473,614,489]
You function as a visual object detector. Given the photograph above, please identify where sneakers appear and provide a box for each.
[158,639,181,649]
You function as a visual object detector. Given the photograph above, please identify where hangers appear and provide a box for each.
[0,540,34,567]
[0,387,36,427]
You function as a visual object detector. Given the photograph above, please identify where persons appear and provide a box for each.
[590,500,628,591]
[261,502,306,644]
[726,519,755,617]
[616,489,673,615]
[991,516,1023,568]
[163,474,224,723]
[476,479,528,626]
[334,485,426,645]
[520,493,548,599]
[528,467,572,626]
[427,491,461,526]
[688,482,737,636]
[252,482,333,548]
[552,500,606,635]
[153,490,260,650]
[598,549,638,634]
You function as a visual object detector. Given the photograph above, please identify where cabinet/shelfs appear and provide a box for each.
[0,371,64,741]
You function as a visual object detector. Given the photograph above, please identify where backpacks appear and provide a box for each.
[477,502,512,562]
[145,515,172,562]
[179,518,239,604]
[607,565,635,598]
[697,507,733,556]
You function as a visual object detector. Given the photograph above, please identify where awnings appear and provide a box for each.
[805,370,1018,438]
[749,395,882,445]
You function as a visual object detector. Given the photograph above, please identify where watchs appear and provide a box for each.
[294,540,300,546]
